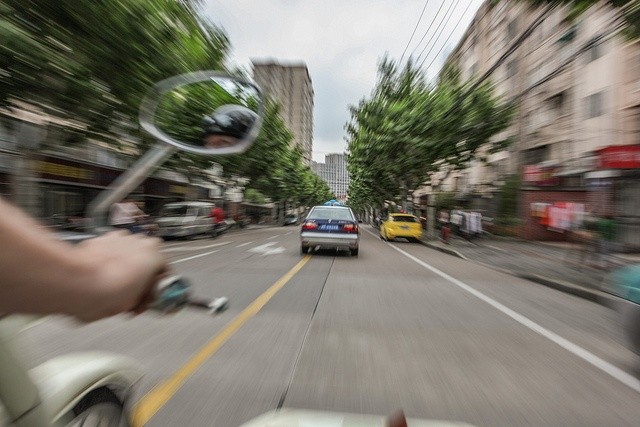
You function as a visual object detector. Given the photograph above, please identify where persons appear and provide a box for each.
[0,199,173,326]
[207,202,226,239]
[439,210,451,243]
[206,103,259,148]
[109,200,152,235]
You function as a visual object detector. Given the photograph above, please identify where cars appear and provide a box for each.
[282,214,298,225]
[379,209,423,243]
[300,200,360,256]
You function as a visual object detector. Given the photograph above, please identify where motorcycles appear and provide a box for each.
[0,70,265,426]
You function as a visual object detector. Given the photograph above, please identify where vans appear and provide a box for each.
[153,200,218,240]
